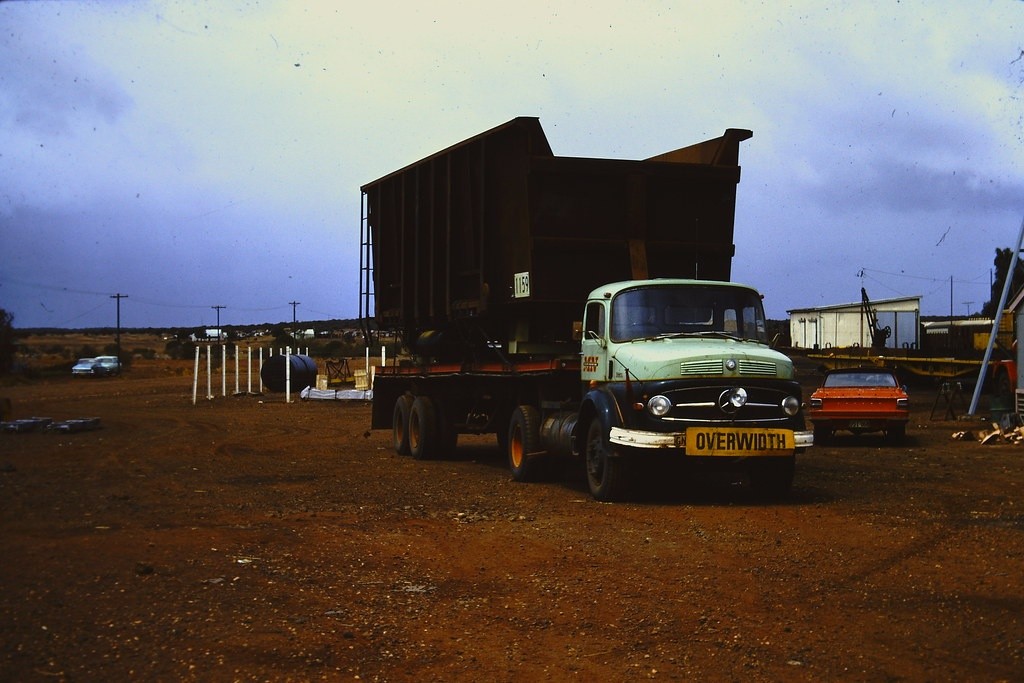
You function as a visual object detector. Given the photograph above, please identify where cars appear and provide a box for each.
[94,355,122,377]
[71,358,101,378]
[809,367,909,444]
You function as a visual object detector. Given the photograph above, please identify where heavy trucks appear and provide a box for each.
[359,117,815,501]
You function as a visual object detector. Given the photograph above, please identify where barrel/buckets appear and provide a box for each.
[261,353,319,393]
[315,374,327,391]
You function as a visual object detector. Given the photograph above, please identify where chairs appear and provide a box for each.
[619,307,659,340]
